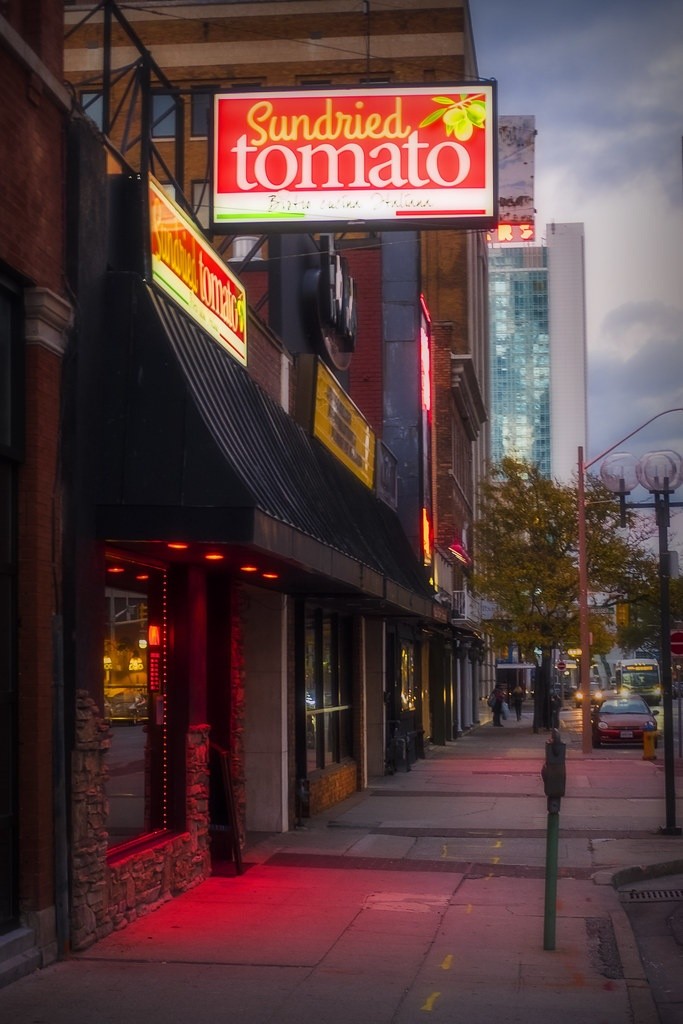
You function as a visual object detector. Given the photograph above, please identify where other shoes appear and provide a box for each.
[520,714,521,716]
[517,717,521,721]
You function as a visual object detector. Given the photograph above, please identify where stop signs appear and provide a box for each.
[556,662,567,672]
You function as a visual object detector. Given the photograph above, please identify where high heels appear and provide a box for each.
[493,722,503,727]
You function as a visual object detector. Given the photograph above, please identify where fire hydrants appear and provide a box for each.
[642,721,662,760]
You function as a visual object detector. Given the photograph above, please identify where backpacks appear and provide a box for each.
[487,693,497,707]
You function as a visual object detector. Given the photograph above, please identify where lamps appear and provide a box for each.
[103,628,147,671]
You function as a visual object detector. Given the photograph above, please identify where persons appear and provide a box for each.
[512,686,526,721]
[492,685,505,727]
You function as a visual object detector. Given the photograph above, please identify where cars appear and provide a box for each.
[591,694,660,749]
[575,682,603,708]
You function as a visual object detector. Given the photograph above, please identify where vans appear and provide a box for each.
[617,659,662,705]
